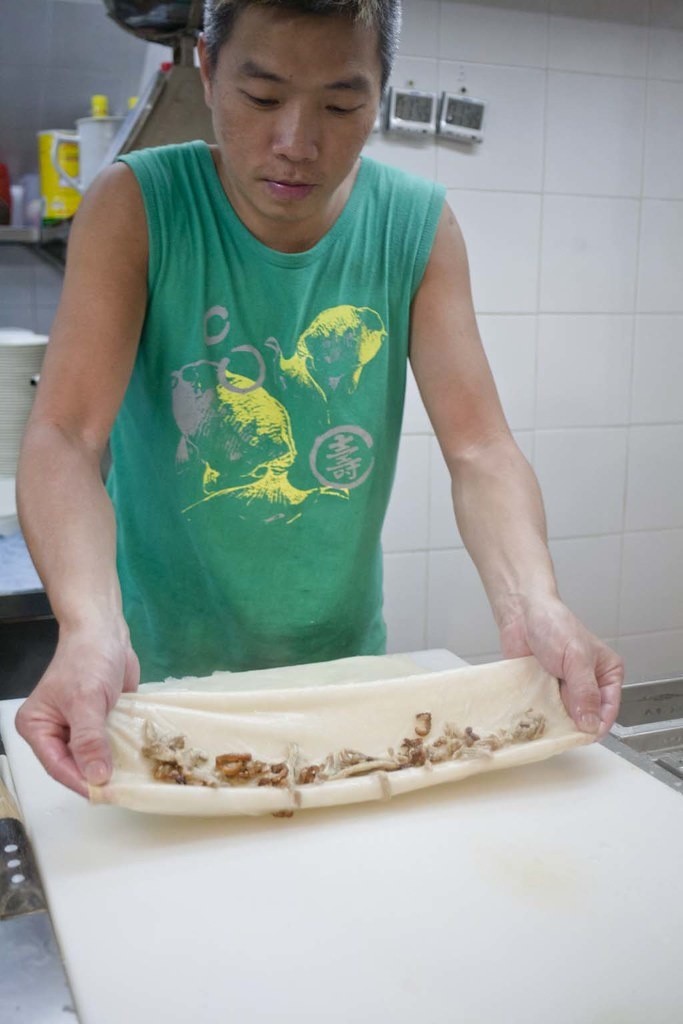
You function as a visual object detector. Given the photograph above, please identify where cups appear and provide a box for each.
[36,129,83,227]
[51,116,125,198]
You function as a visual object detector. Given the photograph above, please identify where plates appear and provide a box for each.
[0,328,49,480]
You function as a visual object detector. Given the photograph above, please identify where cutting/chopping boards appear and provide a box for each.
[0,646,683,1024]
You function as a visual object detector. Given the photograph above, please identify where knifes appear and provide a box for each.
[0,778,81,1024]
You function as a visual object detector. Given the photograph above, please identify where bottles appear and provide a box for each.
[91,95,112,123]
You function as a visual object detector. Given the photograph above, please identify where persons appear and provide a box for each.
[13,1,625,803]
